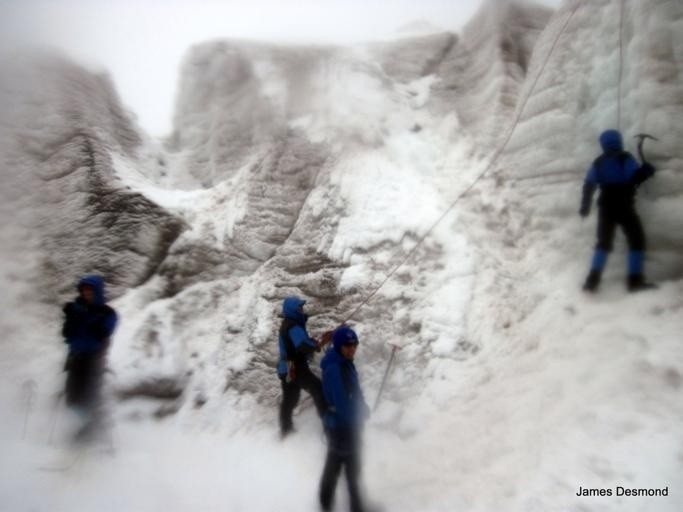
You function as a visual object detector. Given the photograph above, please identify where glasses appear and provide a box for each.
[344,342,356,346]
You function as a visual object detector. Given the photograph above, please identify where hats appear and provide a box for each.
[332,328,357,345]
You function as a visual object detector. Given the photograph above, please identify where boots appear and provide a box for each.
[582,270,601,293]
[629,274,657,292]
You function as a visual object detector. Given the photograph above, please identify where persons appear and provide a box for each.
[278,297,324,436]
[580,131,655,291]
[62,277,117,407]
[318,327,384,510]
